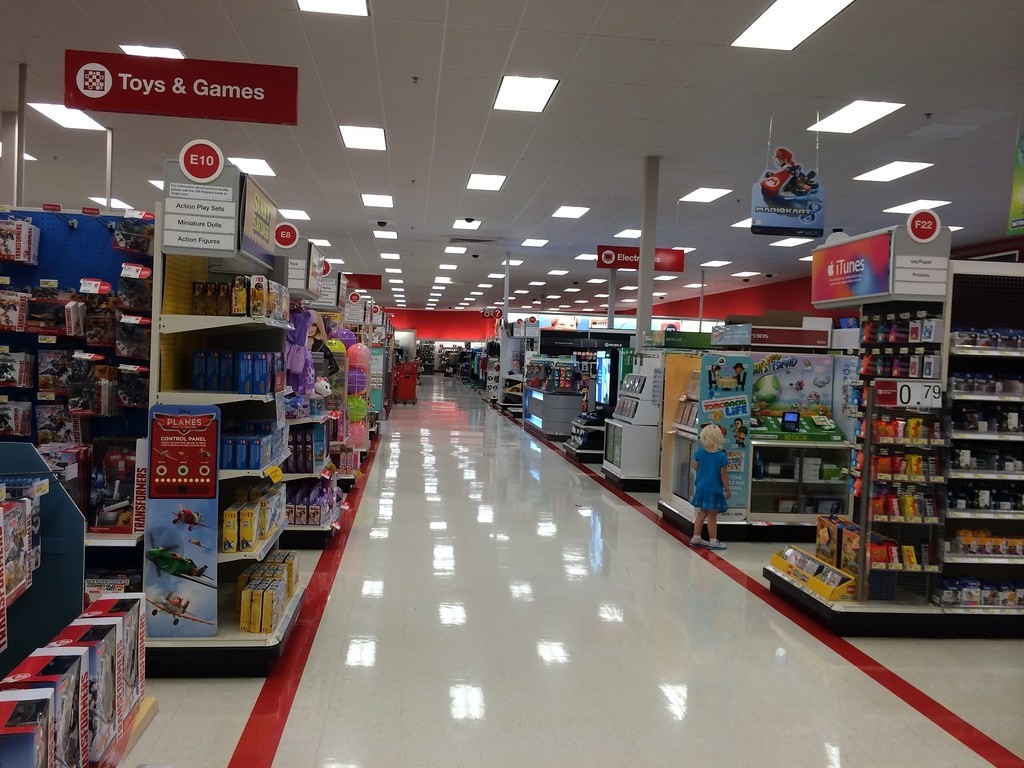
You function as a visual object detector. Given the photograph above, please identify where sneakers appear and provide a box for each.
[689,538,709,547]
[707,540,727,550]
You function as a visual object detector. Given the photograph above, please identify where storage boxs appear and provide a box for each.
[188,272,346,632]
[0,592,146,768]
[816,318,944,603]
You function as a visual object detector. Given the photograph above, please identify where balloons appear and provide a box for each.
[327,329,371,447]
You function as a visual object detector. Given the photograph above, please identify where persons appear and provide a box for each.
[689,425,732,550]
[306,310,339,375]
[446,364,453,374]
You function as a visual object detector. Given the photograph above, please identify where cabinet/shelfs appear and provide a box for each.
[282,296,342,546]
[149,203,306,672]
[330,433,358,488]
[497,373,525,408]
[839,342,941,573]
[939,260,1024,641]
[748,439,855,529]
